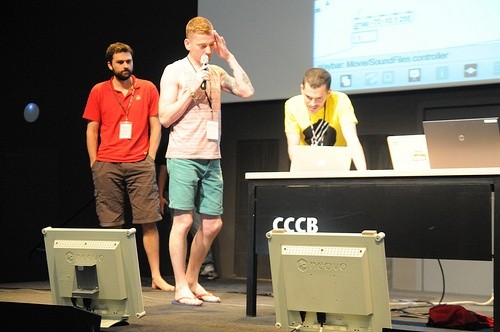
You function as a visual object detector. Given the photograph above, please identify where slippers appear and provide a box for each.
[195,289,222,304]
[172,296,203,306]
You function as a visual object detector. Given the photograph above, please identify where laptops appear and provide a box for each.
[290,145,353,171]
[387,117,500,171]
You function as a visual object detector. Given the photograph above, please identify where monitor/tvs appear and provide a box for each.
[42,226,148,329]
[265,226,392,332]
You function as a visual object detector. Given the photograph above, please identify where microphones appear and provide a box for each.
[200,55,209,90]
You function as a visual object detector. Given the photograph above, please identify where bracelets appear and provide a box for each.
[189,89,194,97]
[148,153,153,154]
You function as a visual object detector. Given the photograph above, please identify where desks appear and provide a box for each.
[243,167,500,332]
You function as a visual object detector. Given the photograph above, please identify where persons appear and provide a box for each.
[156,147,214,275]
[284,68,366,170]
[159,17,255,306]
[82,43,176,291]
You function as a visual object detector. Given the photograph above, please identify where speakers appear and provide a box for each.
[0,301,102,332]
